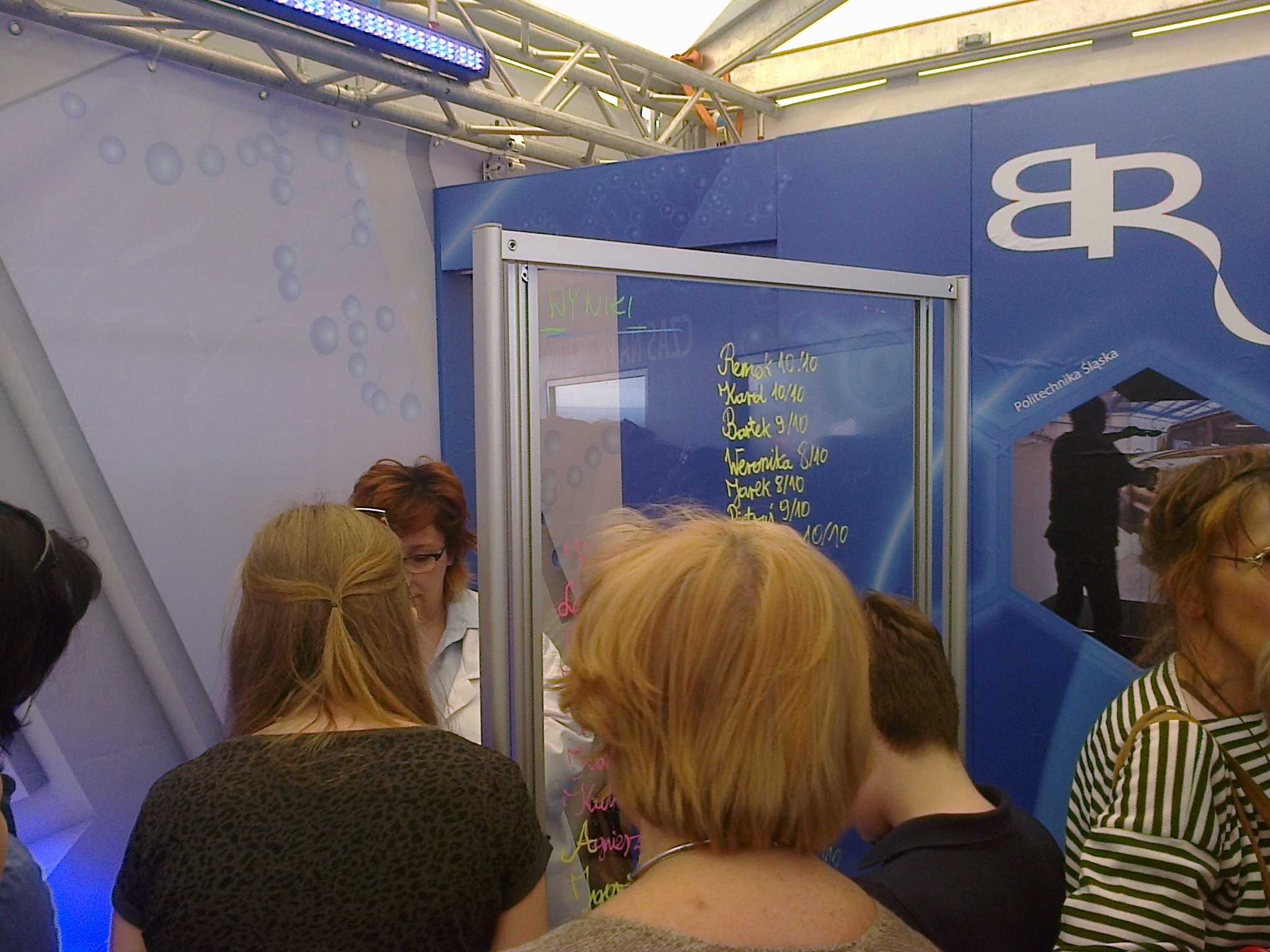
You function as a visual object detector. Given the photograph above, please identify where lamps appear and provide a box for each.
[242,0,491,82]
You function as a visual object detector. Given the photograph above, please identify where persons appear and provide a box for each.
[346,455,595,931]
[839,589,1064,952]
[1044,395,1160,654]
[504,498,946,952]
[0,499,102,952]
[1052,443,1270,952]
[107,501,553,952]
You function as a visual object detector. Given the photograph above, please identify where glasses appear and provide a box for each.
[1208,547,1270,568]
[403,543,447,574]
[1136,429,1163,437]
[352,506,390,530]
[22,508,61,580]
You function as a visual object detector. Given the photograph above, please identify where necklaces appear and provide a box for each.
[1179,650,1270,764]
[627,837,710,885]
